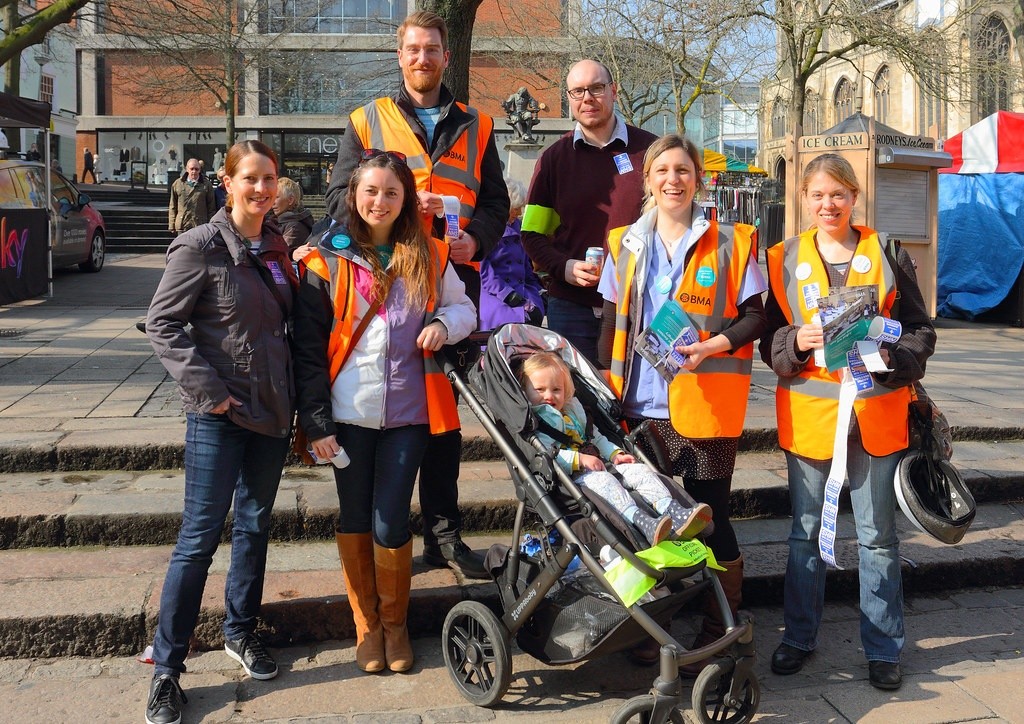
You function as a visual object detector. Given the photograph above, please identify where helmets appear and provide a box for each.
[894,450,977,545]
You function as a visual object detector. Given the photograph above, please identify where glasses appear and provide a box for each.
[358,149,407,168]
[567,81,612,99]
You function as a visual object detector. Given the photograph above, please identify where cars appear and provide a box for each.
[1,159,107,274]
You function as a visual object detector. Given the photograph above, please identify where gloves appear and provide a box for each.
[541,293,550,308]
[504,292,526,307]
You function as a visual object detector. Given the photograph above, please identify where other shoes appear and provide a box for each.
[136,322,146,333]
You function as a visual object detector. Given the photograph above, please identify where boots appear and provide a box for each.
[630,617,672,664]
[373,529,413,671]
[634,508,672,546]
[663,498,713,541]
[678,551,743,678]
[335,525,385,672]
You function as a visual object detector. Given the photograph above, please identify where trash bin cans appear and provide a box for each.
[166,170,180,197]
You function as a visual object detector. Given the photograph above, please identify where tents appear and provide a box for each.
[938,111,1024,319]
[698,147,767,178]
[0,90,53,296]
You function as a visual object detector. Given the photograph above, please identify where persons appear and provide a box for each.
[137,177,314,334]
[168,144,178,167]
[168,159,225,237]
[503,88,538,141]
[26,171,66,259]
[519,60,659,570]
[27,143,41,161]
[326,15,511,577]
[52,159,62,173]
[290,151,477,674]
[212,148,222,172]
[80,148,103,184]
[326,163,334,191]
[818,291,878,338]
[119,146,140,162]
[475,180,543,357]
[145,140,301,724]
[760,154,937,690]
[519,352,714,546]
[597,136,767,675]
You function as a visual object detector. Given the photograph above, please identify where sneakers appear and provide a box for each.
[225,633,278,680]
[144,673,188,724]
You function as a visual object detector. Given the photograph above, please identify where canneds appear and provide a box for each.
[586,247,604,277]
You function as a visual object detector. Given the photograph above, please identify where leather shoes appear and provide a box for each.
[771,642,815,675]
[868,660,902,688]
[423,538,490,578]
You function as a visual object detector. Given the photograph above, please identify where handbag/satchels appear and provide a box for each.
[909,379,953,461]
[293,408,315,466]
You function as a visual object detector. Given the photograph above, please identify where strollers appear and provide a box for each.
[441,320,763,723]
